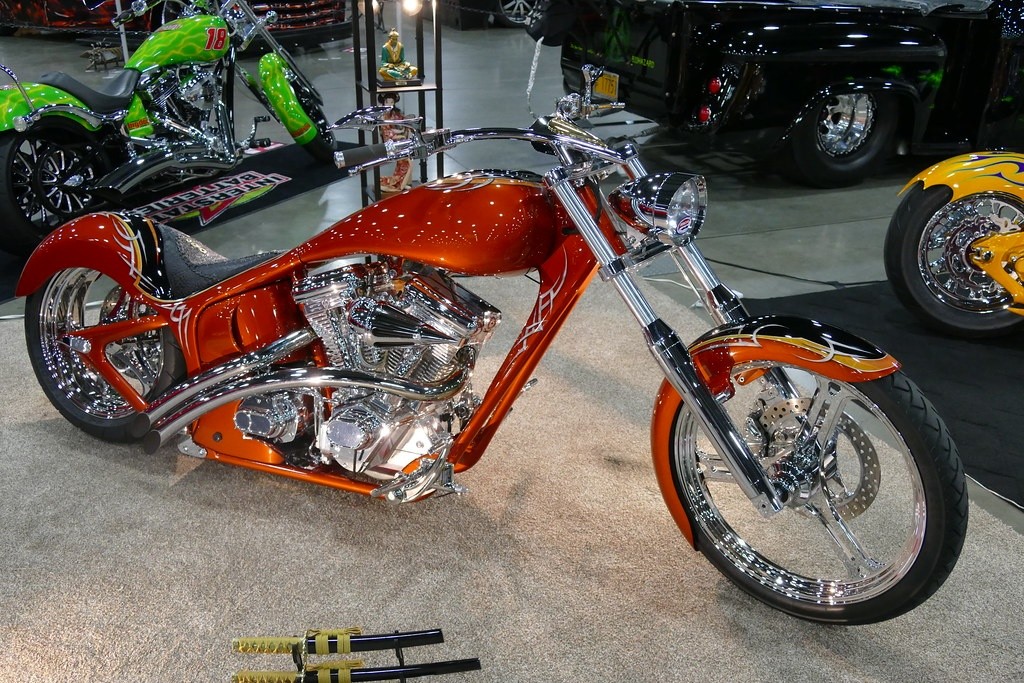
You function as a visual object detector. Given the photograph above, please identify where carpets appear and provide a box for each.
[723,280,1023,511]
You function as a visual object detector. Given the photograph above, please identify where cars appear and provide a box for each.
[560,0,1022,181]
[427,0,550,32]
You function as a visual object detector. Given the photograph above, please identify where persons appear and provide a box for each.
[372,92,413,193]
[379,28,418,81]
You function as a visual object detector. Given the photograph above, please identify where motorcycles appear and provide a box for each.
[18,16,970,629]
[880,149,1024,333]
[0,0,334,255]
